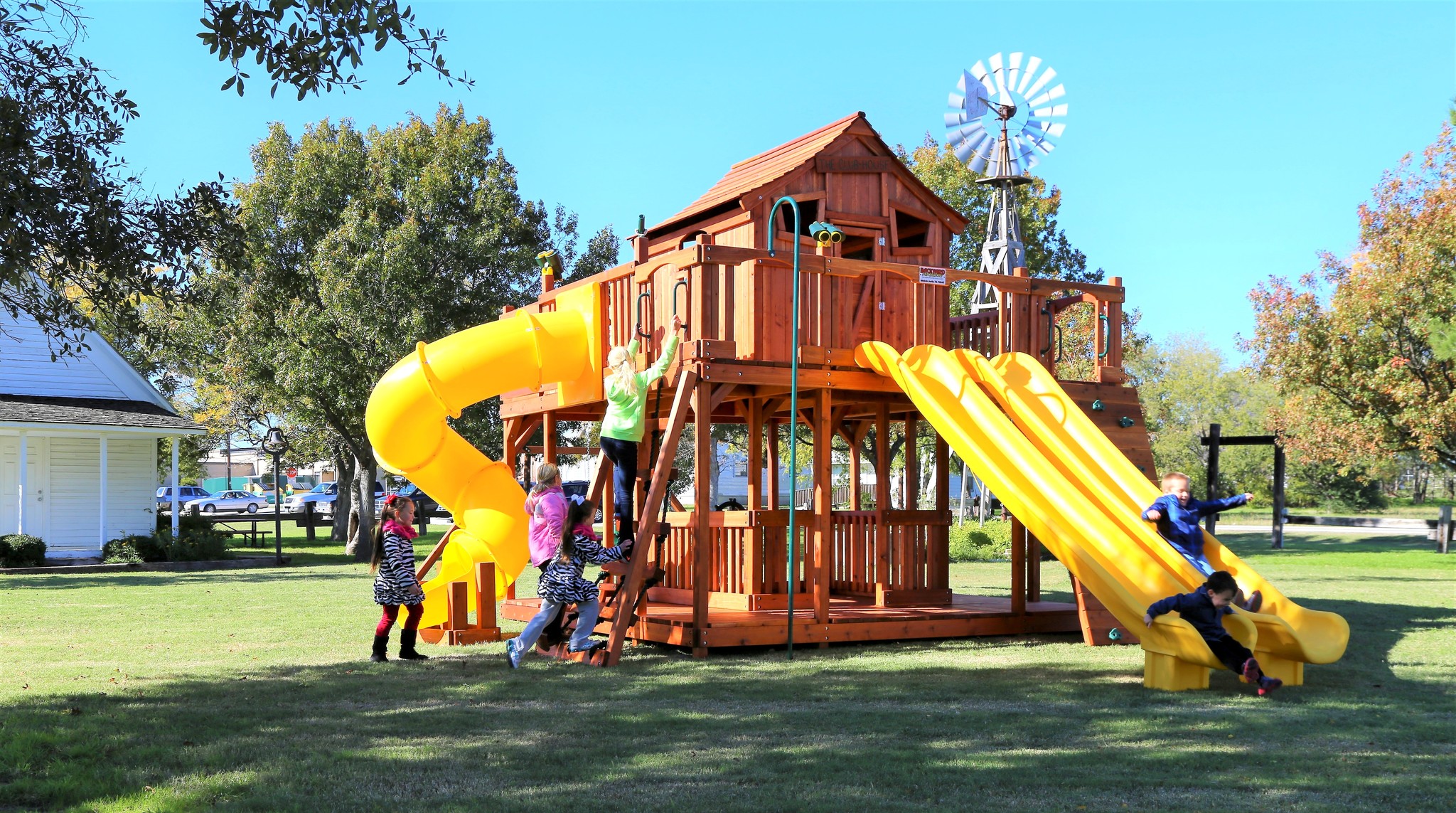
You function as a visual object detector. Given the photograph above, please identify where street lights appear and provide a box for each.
[261,427,290,562]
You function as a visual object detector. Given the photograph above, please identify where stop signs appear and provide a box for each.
[286,467,297,477]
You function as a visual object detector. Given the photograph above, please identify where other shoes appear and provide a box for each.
[615,513,621,520]
[619,557,629,563]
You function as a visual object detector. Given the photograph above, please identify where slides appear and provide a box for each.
[852,340,1258,692]
[365,280,604,632]
[948,347,1351,682]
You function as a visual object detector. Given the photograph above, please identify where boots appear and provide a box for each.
[370,635,389,663]
[399,629,428,660]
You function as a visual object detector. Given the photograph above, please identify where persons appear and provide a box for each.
[1144,570,1283,697]
[506,494,633,669]
[370,494,430,663]
[1142,472,1262,613]
[599,314,681,565]
[525,463,572,652]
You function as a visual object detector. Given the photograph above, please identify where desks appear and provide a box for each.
[213,519,267,548]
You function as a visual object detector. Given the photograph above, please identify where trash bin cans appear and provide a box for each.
[242,483,253,494]
[252,482,283,504]
[286,482,314,497]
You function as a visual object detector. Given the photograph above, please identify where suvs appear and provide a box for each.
[375,483,439,516]
[283,481,385,513]
[517,481,537,492]
[156,485,221,513]
[561,480,602,524]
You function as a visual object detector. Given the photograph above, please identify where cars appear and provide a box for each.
[315,495,387,518]
[183,490,269,514]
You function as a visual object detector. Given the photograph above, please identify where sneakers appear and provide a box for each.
[1259,677,1283,693]
[536,633,550,652]
[1232,589,1243,607]
[1243,591,1262,613]
[548,631,572,647]
[506,638,520,669]
[1245,658,1259,682]
[570,638,600,652]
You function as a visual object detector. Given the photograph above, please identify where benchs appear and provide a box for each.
[230,530,273,548]
